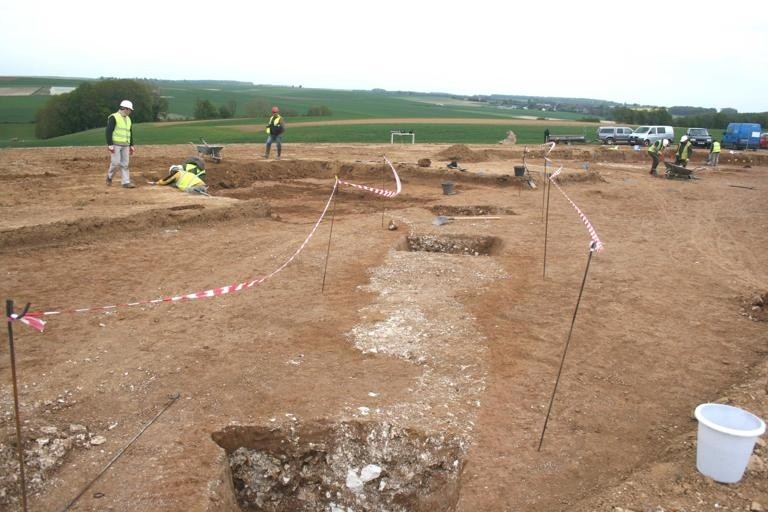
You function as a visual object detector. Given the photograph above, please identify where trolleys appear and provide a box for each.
[661,153,693,180]
[189,136,225,166]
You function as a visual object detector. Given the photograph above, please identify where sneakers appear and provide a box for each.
[106,175,112,187]
[122,182,134,188]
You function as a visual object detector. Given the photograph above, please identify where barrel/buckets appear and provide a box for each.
[441,181,454,195]
[513,165,525,176]
[692,403,766,484]
[634,144,640,150]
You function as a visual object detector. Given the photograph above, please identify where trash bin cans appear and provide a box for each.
[633,145,641,150]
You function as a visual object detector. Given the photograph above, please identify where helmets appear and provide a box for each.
[168,164,179,176]
[118,100,134,111]
[662,139,669,147]
[679,135,688,143]
[272,106,279,114]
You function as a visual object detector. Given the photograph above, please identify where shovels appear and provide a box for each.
[432,216,500,226]
[525,165,537,188]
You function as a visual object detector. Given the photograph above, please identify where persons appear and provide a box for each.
[159,165,208,193]
[544,128,549,143]
[261,106,284,157]
[647,139,668,176]
[710,140,721,164]
[106,100,136,188]
[675,135,692,168]
[177,163,206,180]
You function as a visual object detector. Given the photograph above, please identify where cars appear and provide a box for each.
[596,122,768,151]
